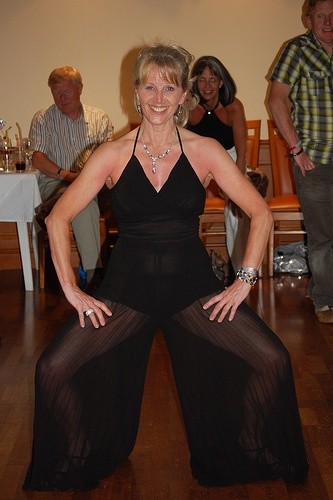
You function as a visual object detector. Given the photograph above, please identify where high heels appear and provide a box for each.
[240,446,288,477]
[38,453,84,490]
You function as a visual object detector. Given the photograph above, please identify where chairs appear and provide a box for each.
[38,119,306,290]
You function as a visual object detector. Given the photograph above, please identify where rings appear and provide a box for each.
[85,309,94,316]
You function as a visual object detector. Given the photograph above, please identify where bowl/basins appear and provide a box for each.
[273,276,309,310]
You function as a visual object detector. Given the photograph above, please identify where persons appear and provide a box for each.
[269,0,333,322]
[27,66,114,284]
[23,45,309,491]
[186,55,251,269]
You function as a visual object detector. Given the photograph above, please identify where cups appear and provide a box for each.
[15,153,27,173]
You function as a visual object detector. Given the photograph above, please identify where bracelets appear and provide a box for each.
[57,168,63,180]
[290,148,303,157]
[291,140,302,151]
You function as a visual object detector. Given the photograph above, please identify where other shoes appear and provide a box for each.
[86,281,101,296]
[315,308,333,322]
[35,203,49,228]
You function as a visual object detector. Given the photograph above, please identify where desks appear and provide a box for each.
[0,170,42,291]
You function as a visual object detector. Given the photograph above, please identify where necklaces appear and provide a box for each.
[141,134,173,174]
[236,267,259,286]
